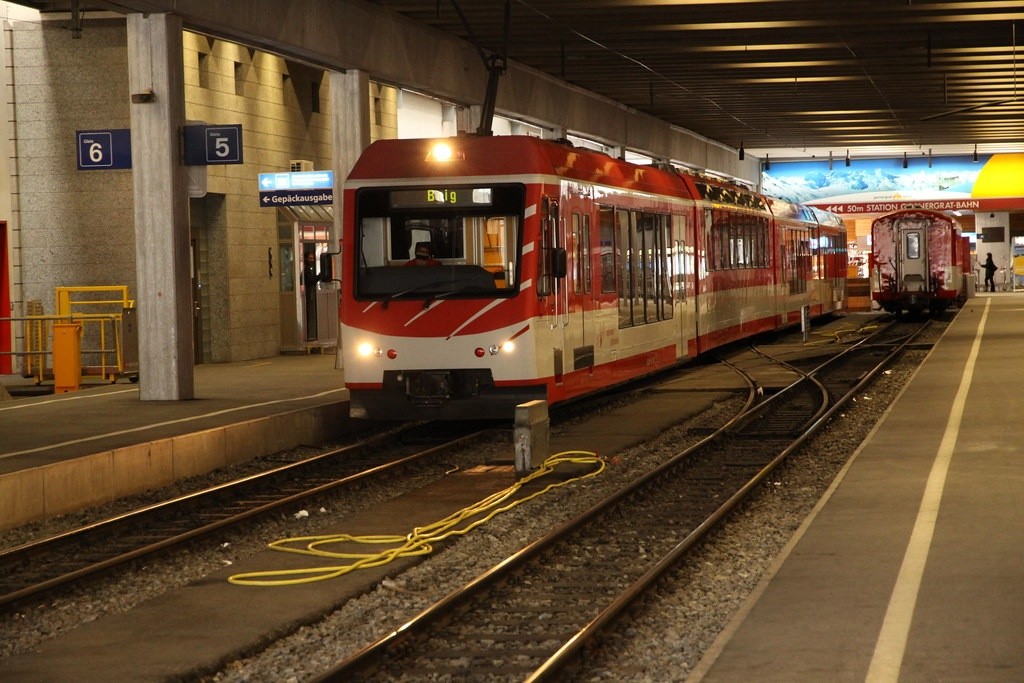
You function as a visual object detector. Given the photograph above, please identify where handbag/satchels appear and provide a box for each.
[993,264,998,272]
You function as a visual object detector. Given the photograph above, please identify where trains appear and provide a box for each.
[321,131,847,419]
[869,209,970,318]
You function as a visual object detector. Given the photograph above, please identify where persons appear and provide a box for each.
[403,242,442,268]
[292,251,322,340]
[979,253,998,292]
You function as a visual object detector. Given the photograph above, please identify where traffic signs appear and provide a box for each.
[258,170,333,190]
[259,188,333,208]
